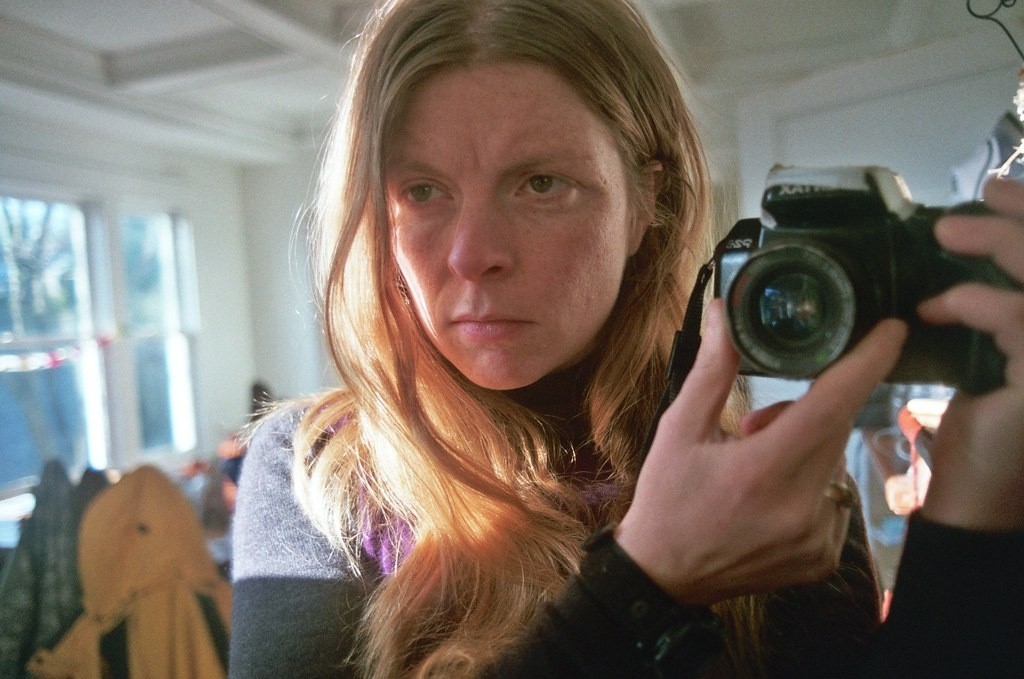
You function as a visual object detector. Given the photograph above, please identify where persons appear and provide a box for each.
[227,0,1024,679]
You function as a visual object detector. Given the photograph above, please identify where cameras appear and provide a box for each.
[712,162,1023,397]
